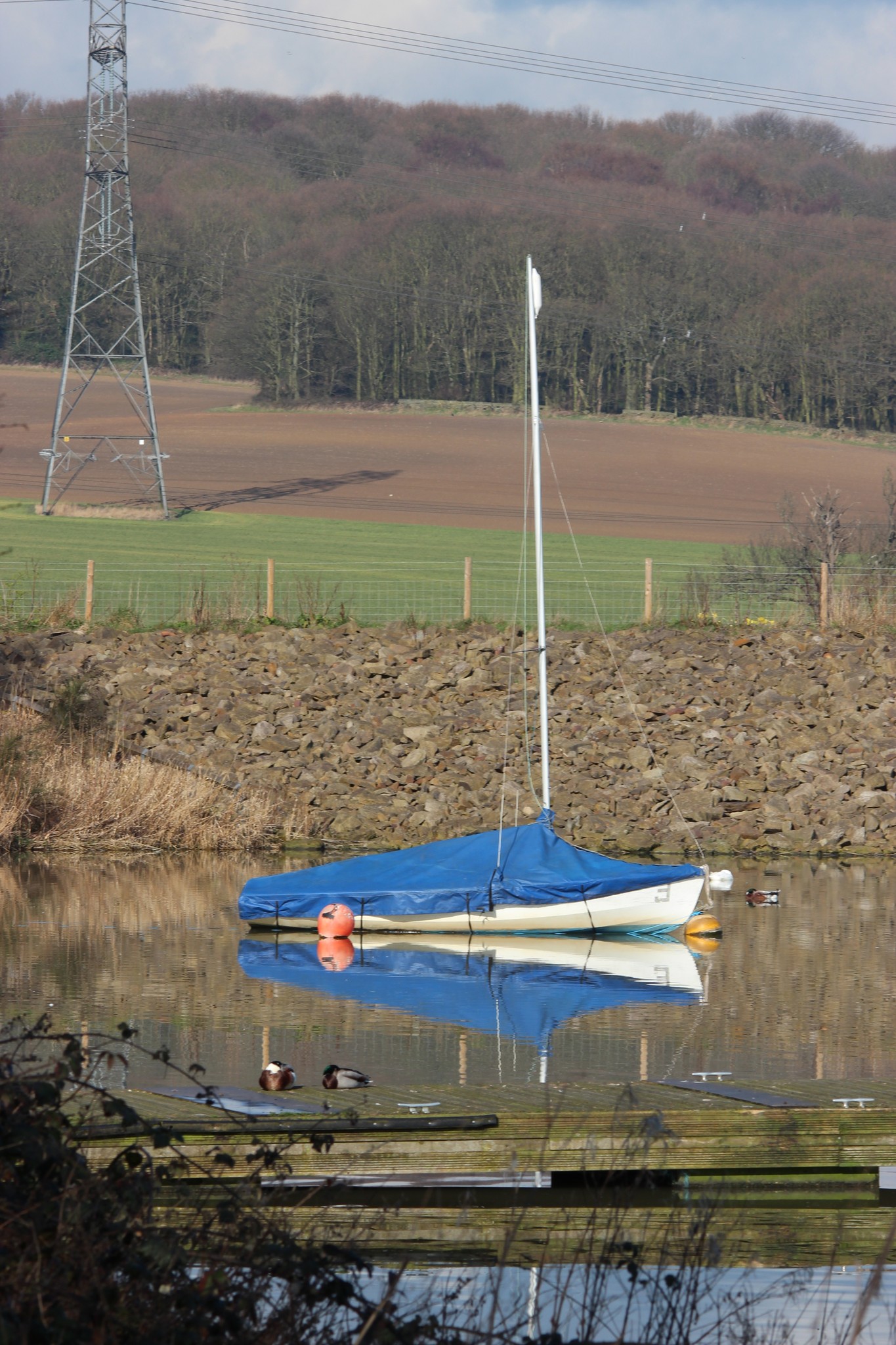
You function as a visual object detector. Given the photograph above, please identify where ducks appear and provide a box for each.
[746,889,781,895]
[321,1065,373,1089]
[258,1061,296,1091]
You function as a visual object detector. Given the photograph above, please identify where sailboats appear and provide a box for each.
[236,924,706,1112]
[240,253,730,937]
